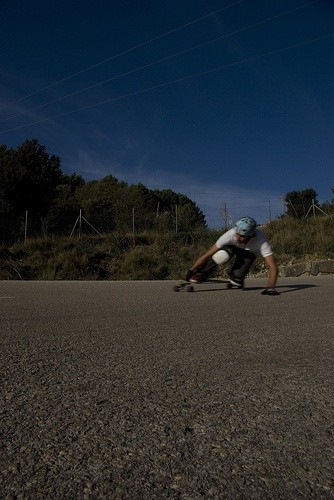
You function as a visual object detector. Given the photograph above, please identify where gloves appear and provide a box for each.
[262,288,280,295]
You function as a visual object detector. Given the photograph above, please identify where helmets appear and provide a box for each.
[235,216,256,237]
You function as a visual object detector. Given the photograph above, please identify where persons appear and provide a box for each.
[183,216,281,296]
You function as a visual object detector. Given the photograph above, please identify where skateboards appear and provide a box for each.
[173,278,244,292]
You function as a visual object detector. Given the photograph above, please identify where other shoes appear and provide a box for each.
[190,275,207,283]
